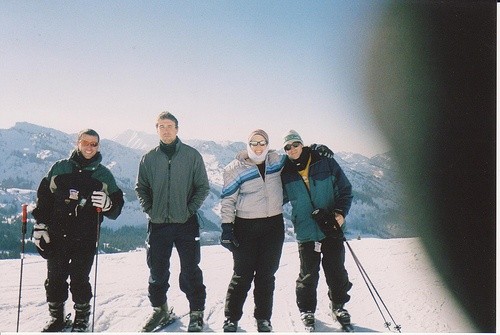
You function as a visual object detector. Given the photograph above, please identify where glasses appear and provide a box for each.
[250,140,267,146]
[79,139,99,147]
[284,141,301,152]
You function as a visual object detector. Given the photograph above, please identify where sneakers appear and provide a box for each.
[332,307,351,325]
[257,319,272,332]
[72,305,92,332]
[299,311,315,326]
[142,308,171,332]
[41,303,65,332]
[223,318,237,332]
[188,314,203,332]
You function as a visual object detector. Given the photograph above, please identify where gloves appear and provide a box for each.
[310,207,342,236]
[31,223,50,251]
[220,222,239,253]
[91,190,112,213]
[235,150,249,162]
[311,144,334,159]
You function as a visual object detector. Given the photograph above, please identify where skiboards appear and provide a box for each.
[40,309,105,332]
[139,305,208,332]
[300,303,355,331]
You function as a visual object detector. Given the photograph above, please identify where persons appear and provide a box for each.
[220,128,334,333]
[33,129,125,333]
[135,111,209,333]
[281,128,354,332]
